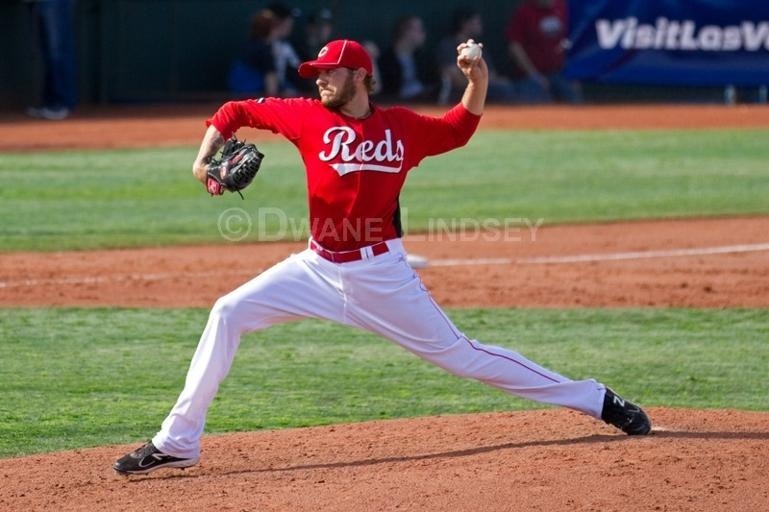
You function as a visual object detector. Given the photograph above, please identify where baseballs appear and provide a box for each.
[458,41,484,63]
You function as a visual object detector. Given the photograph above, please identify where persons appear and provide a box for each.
[110,38,654,475]
[21,2,78,121]
[221,0,585,105]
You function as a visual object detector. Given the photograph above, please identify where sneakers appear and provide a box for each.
[602,386,653,438]
[112,440,201,476]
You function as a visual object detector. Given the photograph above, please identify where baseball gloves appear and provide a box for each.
[204,137,265,195]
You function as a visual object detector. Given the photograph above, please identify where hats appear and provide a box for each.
[295,38,375,84]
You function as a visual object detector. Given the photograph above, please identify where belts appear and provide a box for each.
[309,238,390,265]
